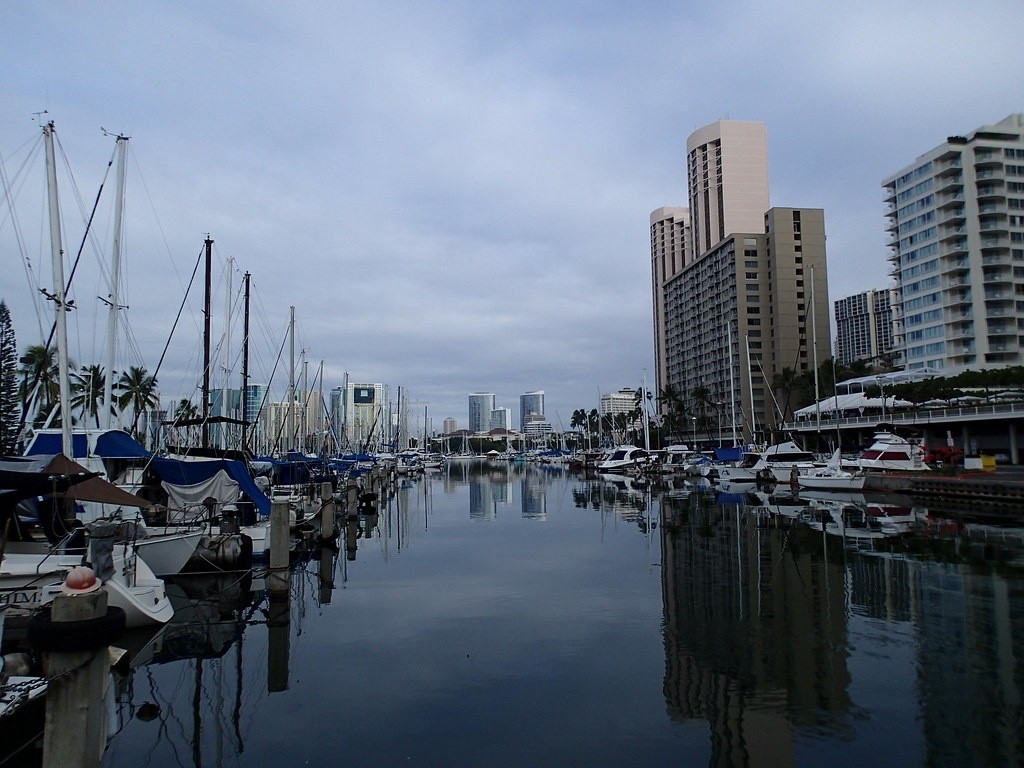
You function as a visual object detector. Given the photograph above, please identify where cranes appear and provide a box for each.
[389,388,428,445]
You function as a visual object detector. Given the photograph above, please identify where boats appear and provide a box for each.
[576,280,931,544]
[0,118,444,646]
[488,410,575,462]
